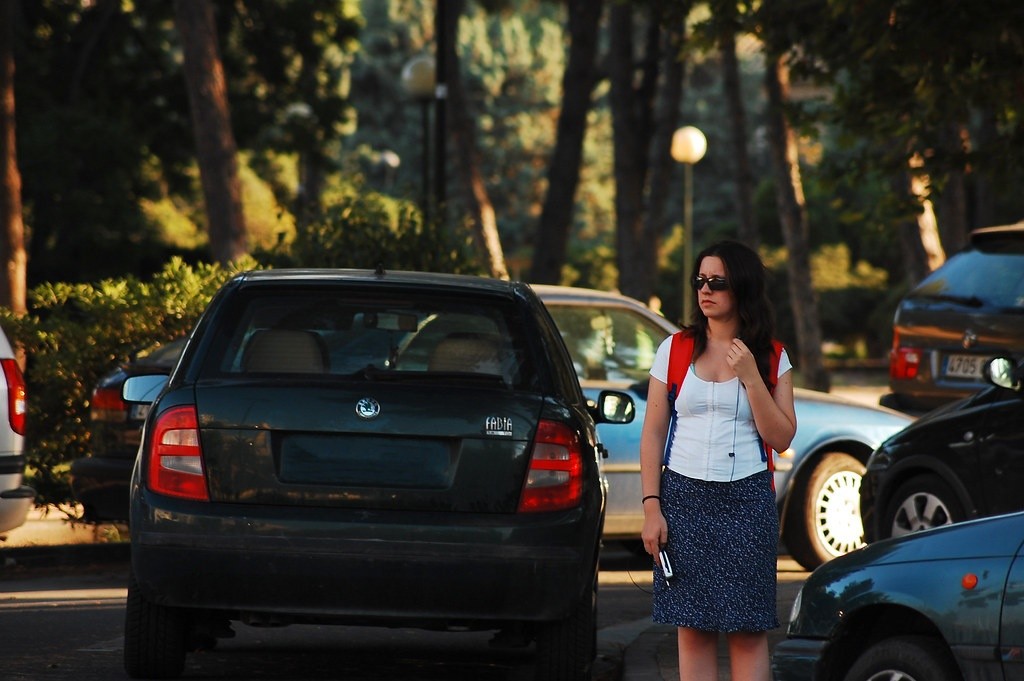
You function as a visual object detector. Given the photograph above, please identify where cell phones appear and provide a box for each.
[658,549,674,586]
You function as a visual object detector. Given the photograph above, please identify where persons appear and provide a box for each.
[641,241,797,681]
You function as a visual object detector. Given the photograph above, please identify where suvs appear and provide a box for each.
[881,215,1023,418]
[122,269,637,681]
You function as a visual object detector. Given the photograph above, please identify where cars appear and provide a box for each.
[393,281,919,574]
[67,327,193,524]
[769,510,1024,681]
[0,324,37,537]
[858,350,1023,550]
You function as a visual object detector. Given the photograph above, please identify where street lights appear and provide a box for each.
[394,54,439,259]
[671,124,708,332]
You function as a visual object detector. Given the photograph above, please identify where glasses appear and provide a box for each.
[696,275,731,291]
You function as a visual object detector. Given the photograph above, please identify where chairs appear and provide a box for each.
[240,331,330,376]
[427,334,508,378]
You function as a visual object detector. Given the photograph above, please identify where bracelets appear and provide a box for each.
[642,495,660,503]
